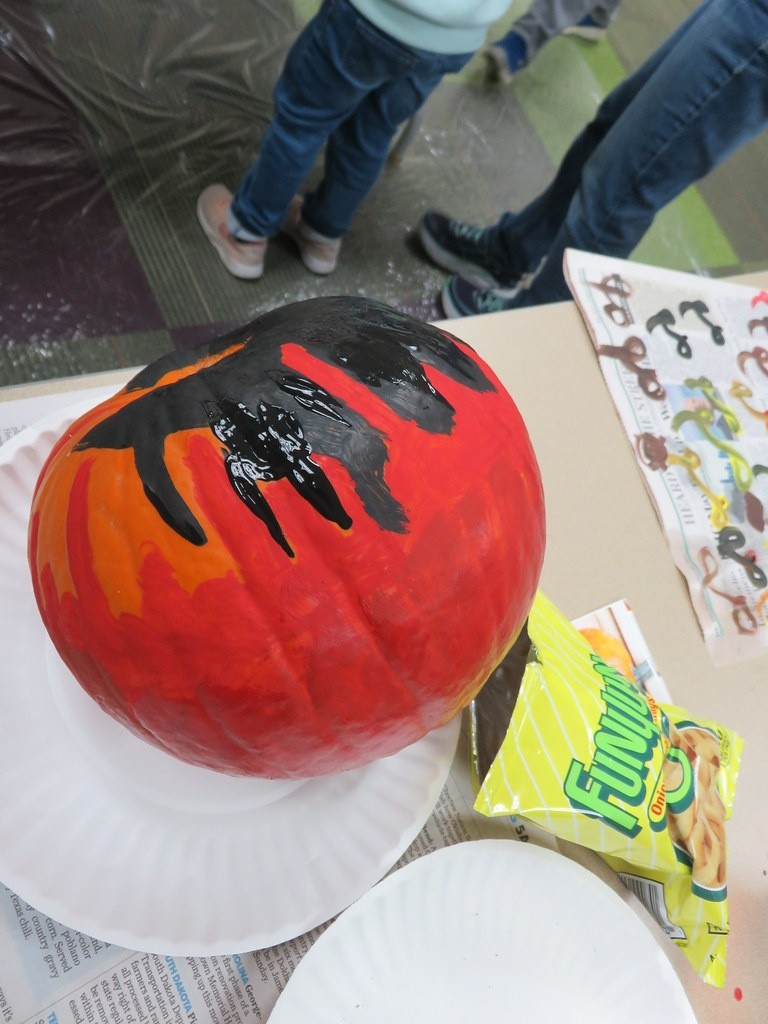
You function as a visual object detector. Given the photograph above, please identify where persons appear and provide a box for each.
[197,0,511,280]
[419,0,768,319]
[484,0,620,88]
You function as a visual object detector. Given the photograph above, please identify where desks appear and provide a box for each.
[0,269,768,1024]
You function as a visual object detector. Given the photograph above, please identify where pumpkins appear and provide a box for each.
[26,295,548,778]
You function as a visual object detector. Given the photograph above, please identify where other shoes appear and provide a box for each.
[562,12,603,42]
[485,33,529,84]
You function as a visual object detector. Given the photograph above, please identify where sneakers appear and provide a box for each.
[277,193,342,275]
[442,273,506,321]
[197,184,266,279]
[420,209,512,291]
